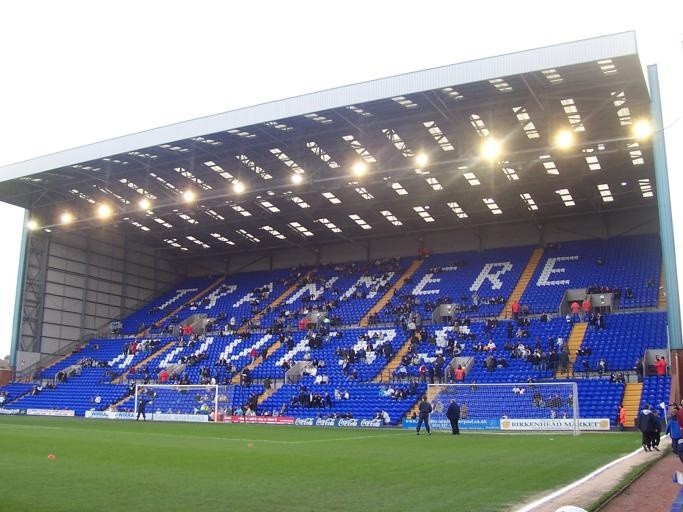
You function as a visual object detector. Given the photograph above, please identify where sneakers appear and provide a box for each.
[643,444,660,452]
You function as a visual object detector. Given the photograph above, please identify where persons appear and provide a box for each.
[597,256,603,265]
[181,282,228,309]
[374,409,391,425]
[504,335,590,374]
[35,369,76,395]
[638,403,682,453]
[412,396,443,435]
[194,357,287,417]
[130,373,153,421]
[403,248,465,285]
[391,291,507,400]
[618,405,625,431]
[276,256,400,304]
[447,399,468,435]
[123,338,161,354]
[266,301,341,384]
[514,377,573,419]
[598,356,666,383]
[504,300,552,337]
[566,297,604,331]
[290,385,354,421]
[110,320,124,335]
[73,344,99,353]
[136,305,180,334]
[240,285,272,339]
[80,357,178,383]
[587,279,655,299]
[176,309,233,393]
[337,299,392,380]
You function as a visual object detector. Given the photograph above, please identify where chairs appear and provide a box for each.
[0,234,673,424]
